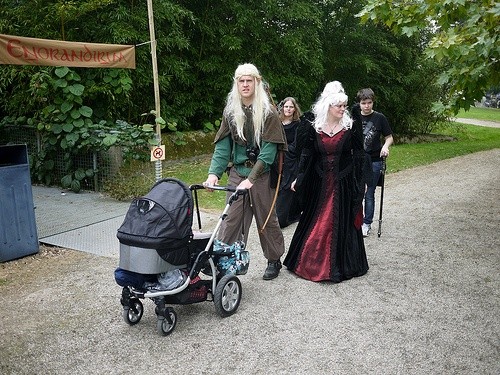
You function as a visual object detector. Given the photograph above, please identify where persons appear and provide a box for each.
[357,88,394,236]
[283,81,369,283]
[276,97,302,229]
[202,63,285,279]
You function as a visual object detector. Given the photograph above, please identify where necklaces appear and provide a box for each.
[329,125,337,136]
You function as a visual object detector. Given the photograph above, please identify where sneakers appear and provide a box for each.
[361,224,372,237]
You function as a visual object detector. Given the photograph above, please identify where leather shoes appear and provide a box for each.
[262,259,283,280]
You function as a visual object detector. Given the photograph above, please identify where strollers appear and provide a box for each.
[114,177,250,337]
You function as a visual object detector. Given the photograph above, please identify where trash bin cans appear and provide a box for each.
[0,143,39,262]
